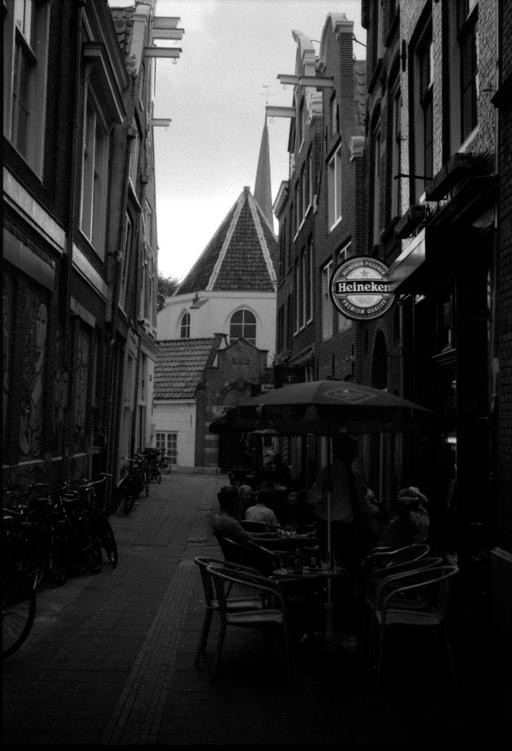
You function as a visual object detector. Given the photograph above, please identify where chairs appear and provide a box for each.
[374,555,444,656]
[364,566,460,689]
[208,565,290,689]
[213,465,461,567]
[194,556,264,670]
[360,541,431,637]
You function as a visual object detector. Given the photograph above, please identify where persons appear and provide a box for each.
[208,458,430,568]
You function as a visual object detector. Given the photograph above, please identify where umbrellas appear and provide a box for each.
[226,374,441,556]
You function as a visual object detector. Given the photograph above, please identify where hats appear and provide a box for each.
[398,486,428,503]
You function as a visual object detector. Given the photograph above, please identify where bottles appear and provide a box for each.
[294,543,304,575]
[309,534,319,570]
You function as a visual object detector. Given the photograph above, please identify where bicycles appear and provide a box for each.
[120,447,171,513]
[1,472,119,646]
